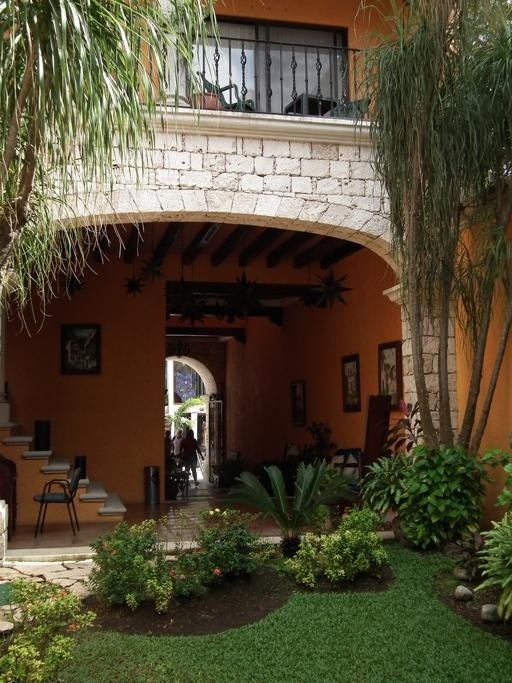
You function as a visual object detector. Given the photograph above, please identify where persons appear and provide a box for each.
[164,429,175,457]
[172,429,183,467]
[179,429,205,485]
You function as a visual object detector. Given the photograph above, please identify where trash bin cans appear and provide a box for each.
[74,456,87,479]
[144,466,160,505]
[34,421,51,451]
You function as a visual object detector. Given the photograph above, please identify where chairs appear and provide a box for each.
[330,99,371,118]
[196,71,256,112]
[33,466,81,537]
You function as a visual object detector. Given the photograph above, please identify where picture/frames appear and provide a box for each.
[341,352,361,413]
[290,379,306,427]
[378,340,402,411]
[61,322,101,375]
